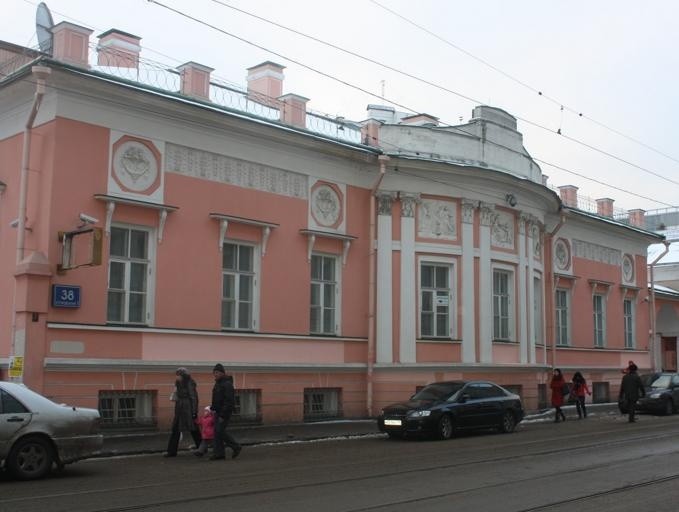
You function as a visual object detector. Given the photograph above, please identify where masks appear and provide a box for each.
[175,376,183,382]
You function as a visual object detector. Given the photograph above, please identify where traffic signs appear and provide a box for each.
[9,355,25,379]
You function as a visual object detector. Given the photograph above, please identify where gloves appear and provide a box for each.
[193,413,197,419]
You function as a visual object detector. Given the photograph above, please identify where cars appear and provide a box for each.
[0,379,106,480]
[618,371,679,416]
[375,377,527,443]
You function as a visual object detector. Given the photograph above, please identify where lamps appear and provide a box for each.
[506,193,517,207]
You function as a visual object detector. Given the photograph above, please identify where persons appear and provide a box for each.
[548,367,567,424]
[191,406,214,456]
[209,364,242,459]
[164,367,208,458]
[569,372,594,420]
[617,361,646,424]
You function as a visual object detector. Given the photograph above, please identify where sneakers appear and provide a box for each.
[163,444,241,460]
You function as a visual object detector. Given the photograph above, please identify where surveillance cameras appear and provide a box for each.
[510,197,517,207]
[645,296,650,302]
[78,213,99,225]
[10,216,27,227]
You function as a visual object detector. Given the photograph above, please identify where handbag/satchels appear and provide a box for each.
[170,392,178,402]
[562,384,580,403]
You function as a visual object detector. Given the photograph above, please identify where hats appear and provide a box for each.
[213,363,225,372]
[204,406,213,415]
